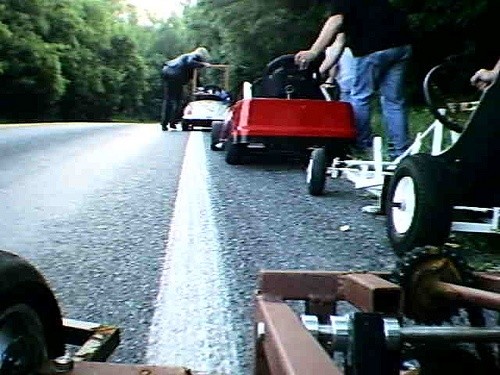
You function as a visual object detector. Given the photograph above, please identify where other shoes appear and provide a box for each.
[162,124,167,131]
[169,124,176,129]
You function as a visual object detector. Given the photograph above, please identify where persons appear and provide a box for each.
[159,47,211,131]
[469,59,500,94]
[294,0,413,162]
